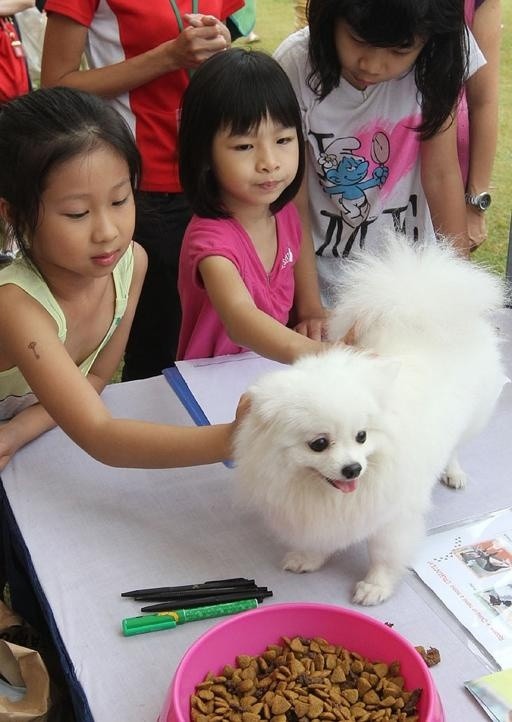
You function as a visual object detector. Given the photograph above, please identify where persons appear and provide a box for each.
[1,2,501,470]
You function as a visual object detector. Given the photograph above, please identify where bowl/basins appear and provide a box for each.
[161,603,445,721]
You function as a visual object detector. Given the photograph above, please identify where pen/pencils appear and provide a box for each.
[120,578,273,637]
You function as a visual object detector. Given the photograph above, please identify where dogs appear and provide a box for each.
[231,225,510,610]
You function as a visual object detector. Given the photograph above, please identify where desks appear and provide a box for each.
[0,306,512,722]
